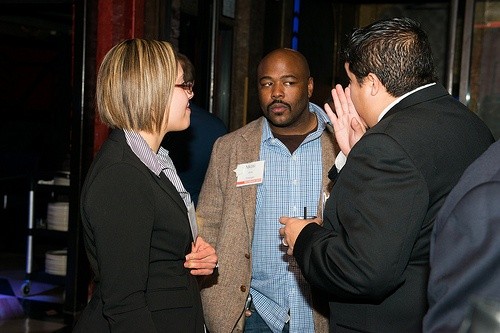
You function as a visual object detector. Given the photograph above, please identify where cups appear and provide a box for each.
[290,216,316,267]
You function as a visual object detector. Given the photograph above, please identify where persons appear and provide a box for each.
[195,47,340,333]
[279,18,496,333]
[71,38,219,333]
[159,53,229,213]
[422,138,500,333]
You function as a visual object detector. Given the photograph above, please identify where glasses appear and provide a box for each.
[175,80,193,91]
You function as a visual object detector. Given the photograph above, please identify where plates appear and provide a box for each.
[45,252,67,276]
[46,202,68,231]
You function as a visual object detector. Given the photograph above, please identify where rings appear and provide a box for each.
[215,263,219,269]
[282,238,288,246]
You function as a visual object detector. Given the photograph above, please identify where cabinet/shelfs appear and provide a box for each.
[19,171,71,300]
